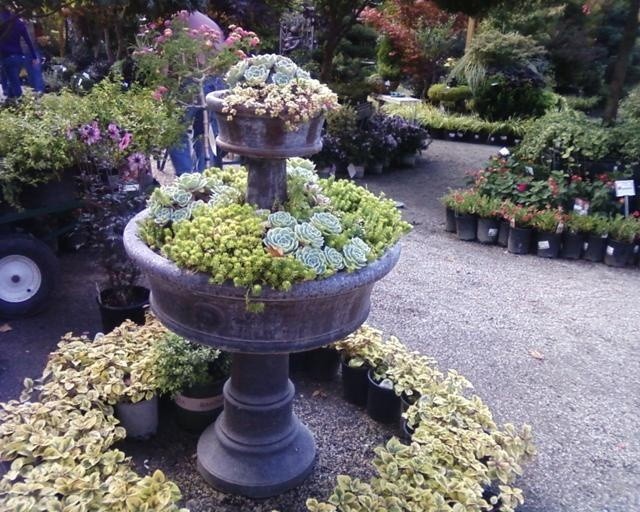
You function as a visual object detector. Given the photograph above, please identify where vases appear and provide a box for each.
[444,151,639,270]
[34,161,78,207]
[96,282,150,333]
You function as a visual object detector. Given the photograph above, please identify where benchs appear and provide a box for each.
[367,92,421,107]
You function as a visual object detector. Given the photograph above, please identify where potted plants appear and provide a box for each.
[1,304,541,510]
[317,112,431,178]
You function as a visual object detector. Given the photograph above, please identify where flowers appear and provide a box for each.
[73,123,153,302]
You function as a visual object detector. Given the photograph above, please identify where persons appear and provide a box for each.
[18,15,45,95]
[159,0,228,177]
[0,7,40,103]
